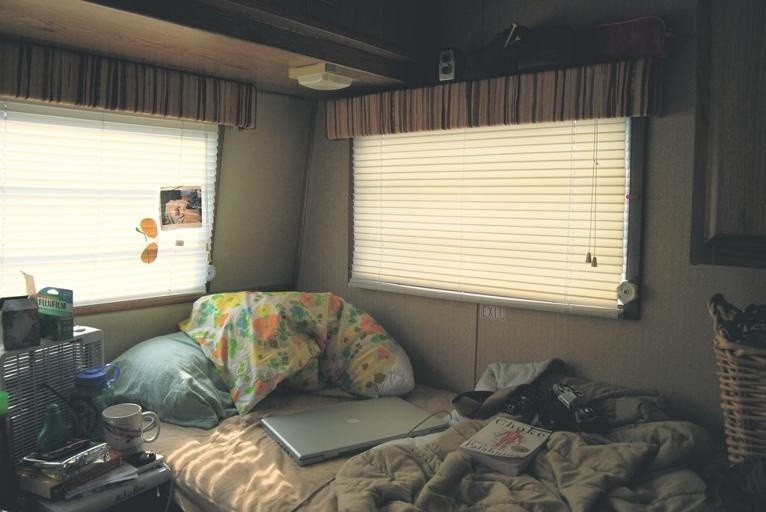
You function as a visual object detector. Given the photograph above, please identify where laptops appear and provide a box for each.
[262,393,451,468]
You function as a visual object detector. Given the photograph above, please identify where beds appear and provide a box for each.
[138,384,725,510]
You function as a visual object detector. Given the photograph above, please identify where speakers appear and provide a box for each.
[438,48,466,81]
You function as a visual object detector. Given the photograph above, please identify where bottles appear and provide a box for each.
[71,363,120,436]
[0,389,18,504]
[33,403,76,455]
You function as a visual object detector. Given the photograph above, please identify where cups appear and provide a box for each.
[101,402,160,457]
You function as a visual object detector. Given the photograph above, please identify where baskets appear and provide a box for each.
[707,292,765,468]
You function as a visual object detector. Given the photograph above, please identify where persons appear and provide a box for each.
[167,194,189,225]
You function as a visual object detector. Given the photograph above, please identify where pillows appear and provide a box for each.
[98,328,237,431]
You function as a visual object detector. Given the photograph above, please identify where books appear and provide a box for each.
[456,413,558,478]
[13,439,139,502]
